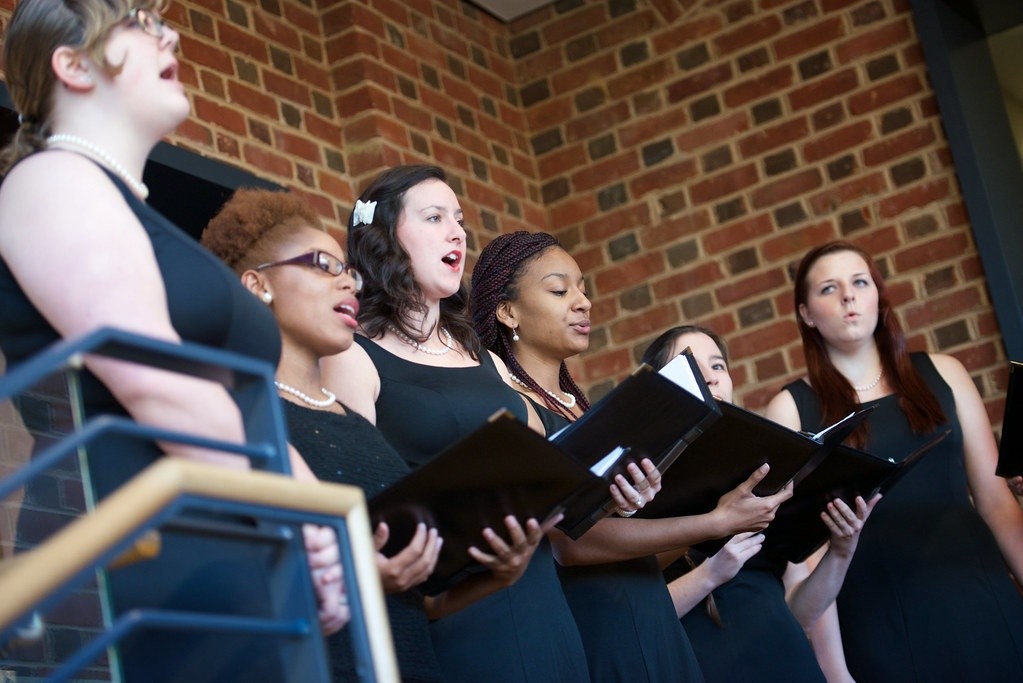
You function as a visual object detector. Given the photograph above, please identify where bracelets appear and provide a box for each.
[614,506,637,517]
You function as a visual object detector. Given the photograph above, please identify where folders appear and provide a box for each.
[547,346,724,540]
[762,427,953,564]
[652,401,882,515]
[365,404,615,598]
[994,361,1023,479]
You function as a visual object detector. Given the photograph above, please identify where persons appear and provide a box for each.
[0,0,351,683]
[468,231,793,683]
[642,324,881,683]
[319,164,662,683]
[765,239,1023,683]
[199,190,543,683]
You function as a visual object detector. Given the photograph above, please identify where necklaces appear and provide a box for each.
[856,366,882,391]
[273,381,336,406]
[388,325,452,354]
[46,134,149,197]
[510,374,575,408]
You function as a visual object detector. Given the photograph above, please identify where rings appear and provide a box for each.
[632,496,642,505]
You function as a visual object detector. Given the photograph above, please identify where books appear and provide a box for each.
[365,409,631,597]
[632,396,951,566]
[994,360,1023,478]
[546,346,722,543]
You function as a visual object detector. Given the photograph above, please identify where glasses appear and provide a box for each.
[93,5,163,39]
[256,250,366,295]
